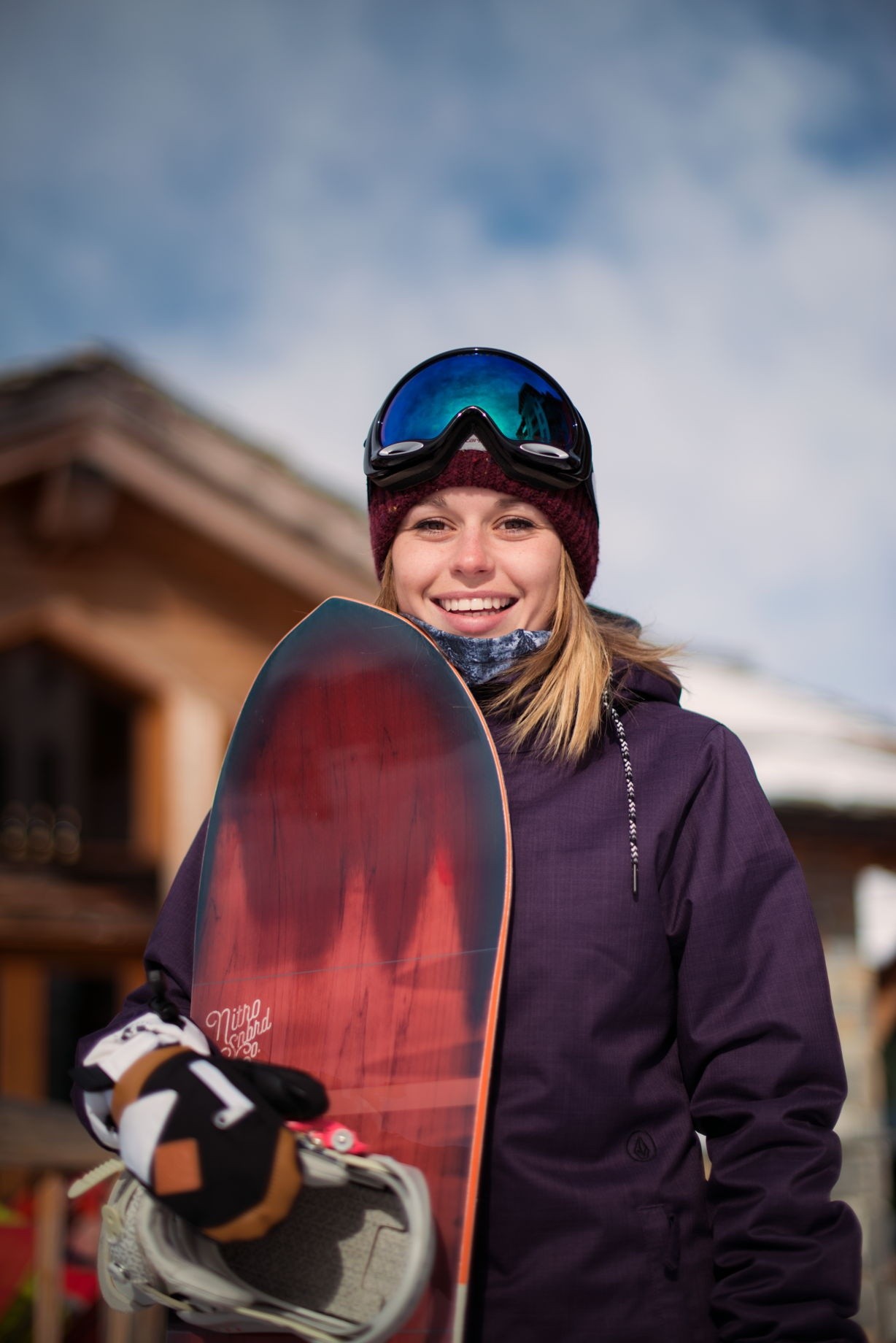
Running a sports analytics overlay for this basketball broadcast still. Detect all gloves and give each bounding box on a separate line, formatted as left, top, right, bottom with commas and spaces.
110, 1040, 330, 1245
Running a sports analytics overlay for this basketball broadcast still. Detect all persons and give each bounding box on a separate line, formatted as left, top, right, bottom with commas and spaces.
63, 347, 876, 1343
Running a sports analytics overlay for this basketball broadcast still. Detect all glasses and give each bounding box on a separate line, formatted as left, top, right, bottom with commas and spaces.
363, 345, 592, 494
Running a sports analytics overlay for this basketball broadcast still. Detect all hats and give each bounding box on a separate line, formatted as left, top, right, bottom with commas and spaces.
368, 433, 599, 601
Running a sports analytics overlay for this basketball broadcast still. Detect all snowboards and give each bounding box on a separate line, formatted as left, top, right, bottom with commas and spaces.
185, 596, 515, 1342
